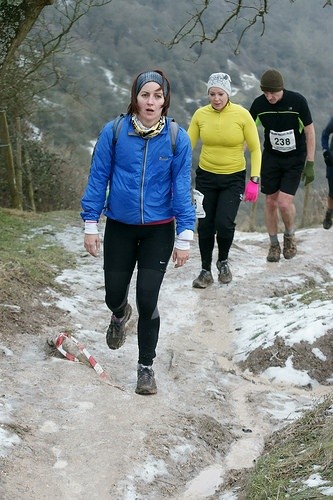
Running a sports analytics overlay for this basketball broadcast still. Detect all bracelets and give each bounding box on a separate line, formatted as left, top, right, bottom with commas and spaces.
250, 176, 260, 184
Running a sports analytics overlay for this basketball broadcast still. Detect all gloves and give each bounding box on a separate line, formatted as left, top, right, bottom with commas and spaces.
301, 161, 314, 186
323, 151, 333, 165
244, 180, 258, 203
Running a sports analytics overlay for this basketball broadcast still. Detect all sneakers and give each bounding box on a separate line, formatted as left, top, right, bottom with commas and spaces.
135, 364, 157, 394
216, 259, 232, 284
106, 304, 132, 349
267, 245, 281, 261
193, 269, 214, 288
283, 233, 297, 259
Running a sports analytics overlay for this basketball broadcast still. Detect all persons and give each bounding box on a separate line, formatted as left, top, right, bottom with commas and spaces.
184, 72, 262, 289
321, 117, 333, 229
80, 69, 196, 395
249, 69, 316, 262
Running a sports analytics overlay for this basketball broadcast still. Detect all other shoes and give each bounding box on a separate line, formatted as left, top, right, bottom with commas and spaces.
323, 211, 333, 229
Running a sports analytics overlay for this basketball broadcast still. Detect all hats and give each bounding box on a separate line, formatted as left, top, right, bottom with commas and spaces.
207, 73, 231, 99
260, 69, 283, 92
